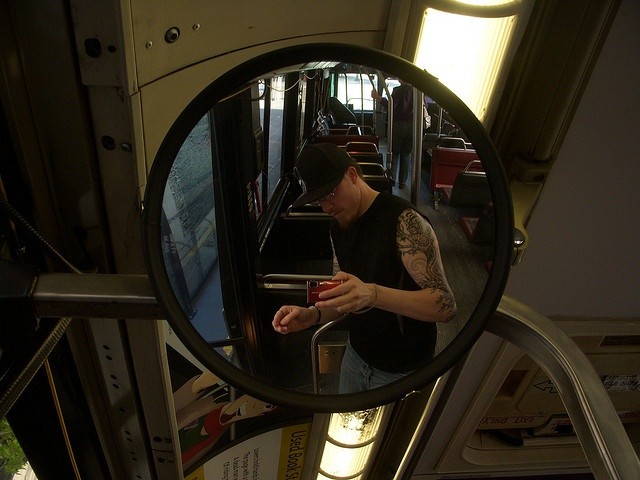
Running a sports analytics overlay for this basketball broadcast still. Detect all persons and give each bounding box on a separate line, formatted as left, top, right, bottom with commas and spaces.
368, 78, 413, 190
272, 141, 460, 395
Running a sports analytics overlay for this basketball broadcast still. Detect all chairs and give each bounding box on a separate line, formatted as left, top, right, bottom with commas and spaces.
456, 207, 492, 245
352, 174, 393, 196
345, 141, 377, 152
422, 133, 449, 150
441, 159, 491, 209
347, 150, 383, 164
343, 161, 384, 174
437, 137, 473, 149
279, 199, 334, 226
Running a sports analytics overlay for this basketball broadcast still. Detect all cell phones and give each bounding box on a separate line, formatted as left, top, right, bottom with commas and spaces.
306, 278, 354, 305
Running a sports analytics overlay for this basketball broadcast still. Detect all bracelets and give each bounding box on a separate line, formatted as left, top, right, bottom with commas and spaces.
307, 305, 322, 325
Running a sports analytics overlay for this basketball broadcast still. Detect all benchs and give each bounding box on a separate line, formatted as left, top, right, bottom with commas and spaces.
254, 263, 334, 331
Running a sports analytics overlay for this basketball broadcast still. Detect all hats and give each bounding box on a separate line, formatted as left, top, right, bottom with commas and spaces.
292, 137, 350, 210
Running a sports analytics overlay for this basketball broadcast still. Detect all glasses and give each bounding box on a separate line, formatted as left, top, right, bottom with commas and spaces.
311, 188, 336, 203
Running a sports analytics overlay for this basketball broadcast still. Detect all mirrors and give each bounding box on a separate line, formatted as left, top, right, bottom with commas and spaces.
141, 42, 514, 413
